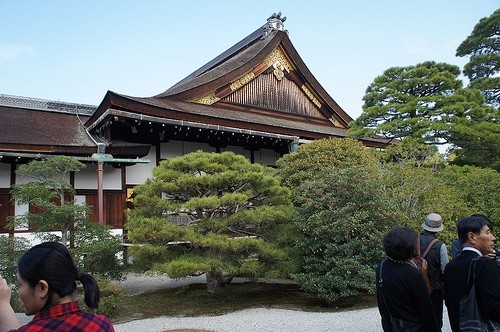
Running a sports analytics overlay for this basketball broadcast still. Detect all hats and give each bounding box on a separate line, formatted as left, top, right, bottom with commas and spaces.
421, 213, 444, 233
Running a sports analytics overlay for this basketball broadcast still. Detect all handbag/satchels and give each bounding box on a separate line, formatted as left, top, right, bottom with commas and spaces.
457, 285, 495, 332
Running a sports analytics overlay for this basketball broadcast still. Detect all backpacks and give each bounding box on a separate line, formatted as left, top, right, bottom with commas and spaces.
410, 231, 440, 294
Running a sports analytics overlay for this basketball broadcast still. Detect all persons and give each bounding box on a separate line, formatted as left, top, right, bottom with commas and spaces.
414, 213, 449, 332
375, 228, 435, 332
442, 214, 500, 332
0, 242, 115, 332
